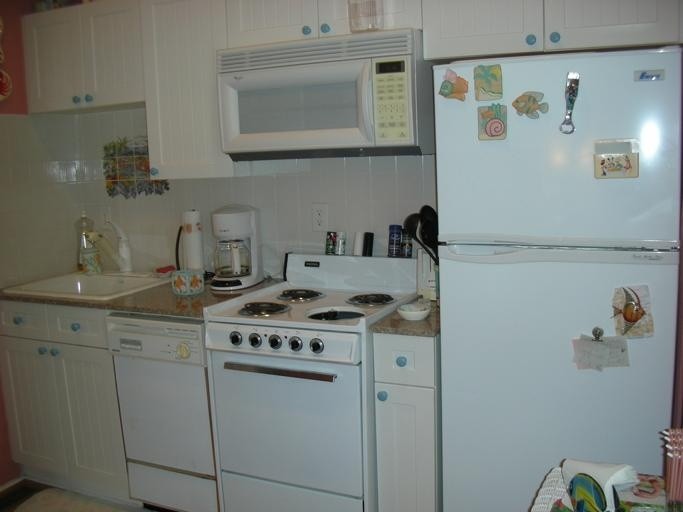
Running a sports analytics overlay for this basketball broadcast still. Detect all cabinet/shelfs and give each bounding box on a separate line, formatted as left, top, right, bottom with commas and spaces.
369, 326, 441, 512
0, 296, 142, 508
225, 0, 422, 47
421, 0, 683, 61
21, 0, 145, 115
139, 0, 309, 181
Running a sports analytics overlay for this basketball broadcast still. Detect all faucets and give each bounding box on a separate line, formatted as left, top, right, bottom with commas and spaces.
86, 220, 131, 274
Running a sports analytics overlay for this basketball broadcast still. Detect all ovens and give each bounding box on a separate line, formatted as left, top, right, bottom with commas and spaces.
206, 321, 364, 512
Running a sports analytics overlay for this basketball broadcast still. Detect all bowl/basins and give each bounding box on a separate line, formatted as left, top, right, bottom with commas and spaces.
430, 48, 681, 510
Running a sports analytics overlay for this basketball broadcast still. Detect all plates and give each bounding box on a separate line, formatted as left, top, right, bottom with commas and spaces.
396, 303, 431, 321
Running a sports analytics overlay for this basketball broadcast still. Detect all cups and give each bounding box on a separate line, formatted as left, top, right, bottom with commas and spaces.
80, 248, 102, 274
347, 0, 383, 32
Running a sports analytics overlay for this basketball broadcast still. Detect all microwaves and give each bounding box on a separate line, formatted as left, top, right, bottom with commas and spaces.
215, 31, 427, 158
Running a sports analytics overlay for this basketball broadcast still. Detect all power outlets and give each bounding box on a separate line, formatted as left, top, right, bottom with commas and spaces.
312, 204, 328, 231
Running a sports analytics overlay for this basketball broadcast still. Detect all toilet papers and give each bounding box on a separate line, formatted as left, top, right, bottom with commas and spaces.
179, 211, 203, 269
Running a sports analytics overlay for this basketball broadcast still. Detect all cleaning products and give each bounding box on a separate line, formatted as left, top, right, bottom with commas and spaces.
75, 207, 95, 270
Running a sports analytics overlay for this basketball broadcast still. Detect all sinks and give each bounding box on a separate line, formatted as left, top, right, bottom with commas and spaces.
2, 270, 173, 302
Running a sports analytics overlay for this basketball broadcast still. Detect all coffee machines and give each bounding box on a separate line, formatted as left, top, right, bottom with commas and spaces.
211, 204, 264, 289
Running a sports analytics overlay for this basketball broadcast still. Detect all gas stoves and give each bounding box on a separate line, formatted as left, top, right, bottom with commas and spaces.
205, 284, 418, 365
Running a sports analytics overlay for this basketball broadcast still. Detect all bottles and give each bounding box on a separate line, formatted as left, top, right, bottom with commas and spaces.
325, 224, 411, 258
181, 211, 204, 274
76, 210, 97, 268
